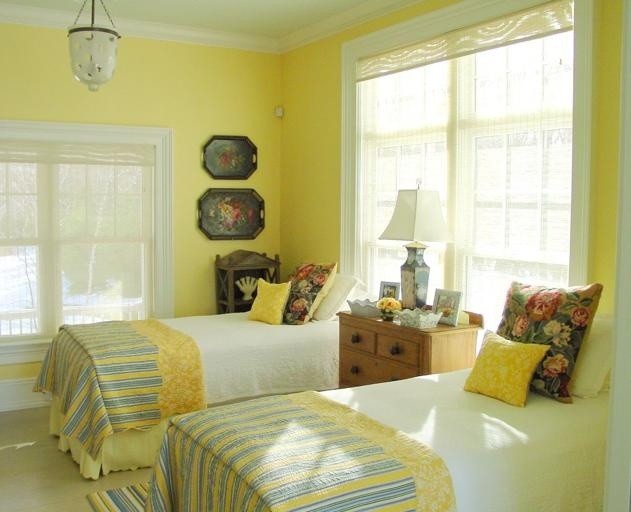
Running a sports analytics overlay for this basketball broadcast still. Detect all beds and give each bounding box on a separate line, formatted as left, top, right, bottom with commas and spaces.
145, 367, 612, 511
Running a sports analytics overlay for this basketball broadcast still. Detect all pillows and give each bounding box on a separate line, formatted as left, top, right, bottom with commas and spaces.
247, 261, 362, 324
463, 280, 617, 406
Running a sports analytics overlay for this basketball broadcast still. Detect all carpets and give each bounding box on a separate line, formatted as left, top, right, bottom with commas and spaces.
85, 481, 151, 512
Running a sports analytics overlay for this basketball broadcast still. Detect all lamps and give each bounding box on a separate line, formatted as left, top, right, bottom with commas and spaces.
68, 1, 123, 91
376, 177, 451, 309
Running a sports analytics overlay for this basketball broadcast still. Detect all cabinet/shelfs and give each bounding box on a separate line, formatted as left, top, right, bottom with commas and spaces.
214, 250, 282, 315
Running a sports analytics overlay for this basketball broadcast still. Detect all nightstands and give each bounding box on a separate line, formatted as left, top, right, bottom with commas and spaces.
335, 310, 486, 390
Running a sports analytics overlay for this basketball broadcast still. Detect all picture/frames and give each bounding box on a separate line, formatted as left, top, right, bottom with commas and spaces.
196, 136, 266, 240
377, 280, 464, 327
